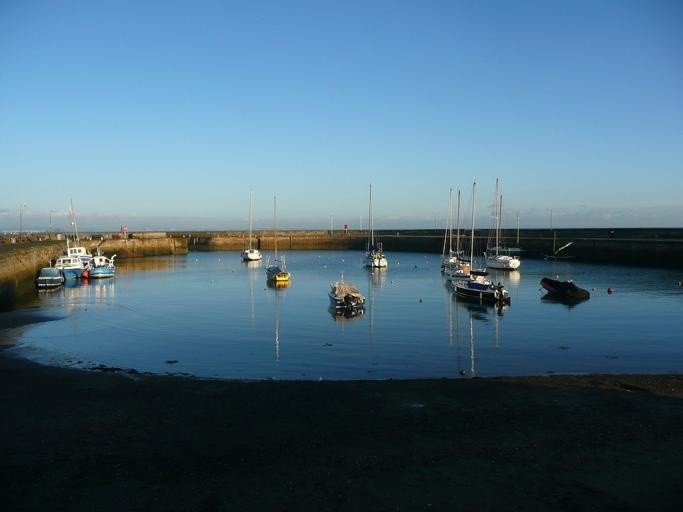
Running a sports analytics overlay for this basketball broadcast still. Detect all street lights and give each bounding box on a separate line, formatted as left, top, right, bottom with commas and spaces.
18, 202, 29, 244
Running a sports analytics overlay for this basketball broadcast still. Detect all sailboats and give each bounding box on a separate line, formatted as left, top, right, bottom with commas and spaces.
366, 266, 388, 350
362, 183, 389, 268
242, 185, 264, 261
265, 278, 291, 370
438, 175, 592, 307
444, 281, 501, 378
265, 192, 292, 281
246, 261, 261, 329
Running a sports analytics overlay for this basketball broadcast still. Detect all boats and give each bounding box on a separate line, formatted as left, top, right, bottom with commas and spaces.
327, 273, 364, 307
327, 303, 366, 331
34, 197, 118, 291
